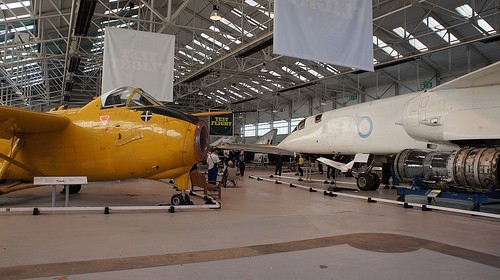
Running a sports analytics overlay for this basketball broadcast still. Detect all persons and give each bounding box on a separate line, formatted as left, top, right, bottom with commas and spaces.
206, 148, 222, 191
219, 149, 247, 189
190, 161, 203, 173
293, 153, 399, 189
275, 152, 283, 178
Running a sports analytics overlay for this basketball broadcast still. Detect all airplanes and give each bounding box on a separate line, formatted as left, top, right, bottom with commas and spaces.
209, 61, 500, 191
0, 86, 232, 205
